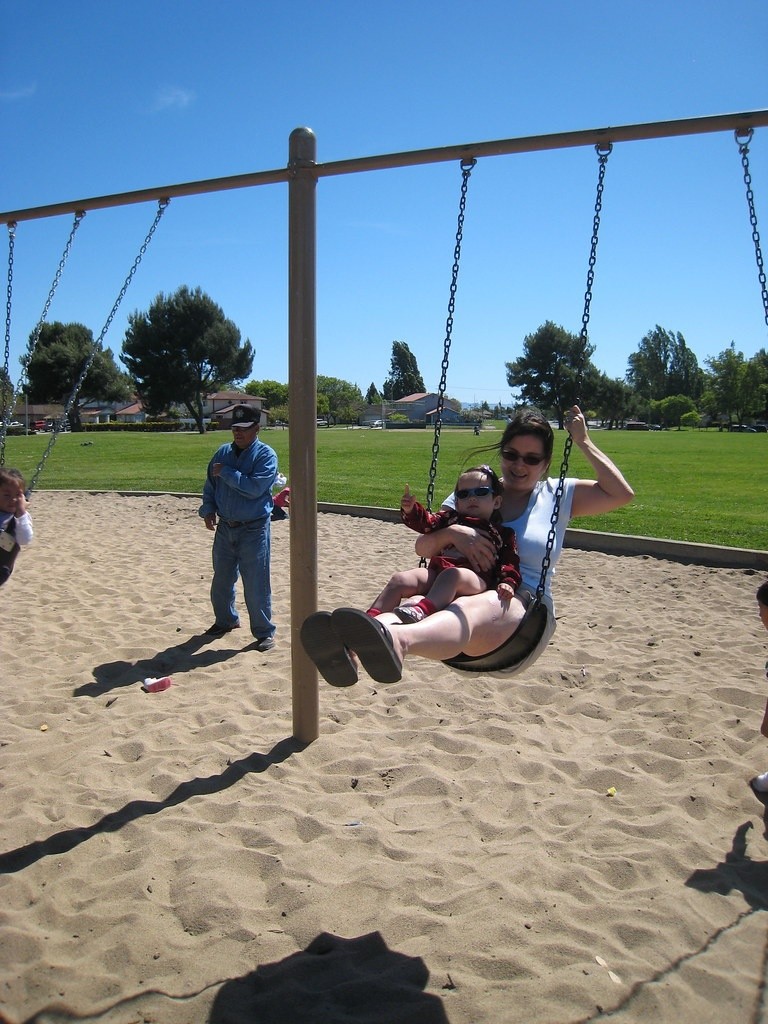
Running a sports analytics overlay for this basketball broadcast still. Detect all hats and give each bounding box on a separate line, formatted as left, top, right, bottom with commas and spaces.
232, 404, 261, 427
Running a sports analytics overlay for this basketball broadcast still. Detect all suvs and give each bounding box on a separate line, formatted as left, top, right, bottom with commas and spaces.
750, 425, 767, 432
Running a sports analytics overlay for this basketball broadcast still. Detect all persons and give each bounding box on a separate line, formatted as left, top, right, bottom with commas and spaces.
300, 405, 634, 687
198, 405, 278, 650
271, 487, 290, 521
365, 465, 522, 626
473, 427, 480, 435
507, 415, 512, 424
0, 467, 33, 586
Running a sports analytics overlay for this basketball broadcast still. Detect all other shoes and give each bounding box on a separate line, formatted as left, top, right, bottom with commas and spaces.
204, 620, 239, 638
144, 676, 171, 693
257, 636, 275, 652
752, 772, 768, 792
393, 603, 427, 624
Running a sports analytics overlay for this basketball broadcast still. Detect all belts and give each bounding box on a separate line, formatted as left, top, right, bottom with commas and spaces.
220, 516, 263, 527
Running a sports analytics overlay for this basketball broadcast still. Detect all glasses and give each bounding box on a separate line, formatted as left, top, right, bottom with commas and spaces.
502, 446, 547, 465
455, 487, 495, 500
232, 423, 256, 432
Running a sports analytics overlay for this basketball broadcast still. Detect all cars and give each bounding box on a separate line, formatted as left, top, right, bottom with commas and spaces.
370, 420, 384, 428
9, 422, 23, 427
0, 422, 8, 426
651, 425, 660, 430
732, 425, 756, 432
317, 419, 327, 426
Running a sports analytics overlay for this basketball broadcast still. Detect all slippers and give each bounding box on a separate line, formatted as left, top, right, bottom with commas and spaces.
300, 611, 359, 687
332, 607, 402, 683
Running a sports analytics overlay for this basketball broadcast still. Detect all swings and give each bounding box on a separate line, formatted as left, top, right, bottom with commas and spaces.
0, 193, 173, 573
732, 127, 768, 328
415, 139, 618, 675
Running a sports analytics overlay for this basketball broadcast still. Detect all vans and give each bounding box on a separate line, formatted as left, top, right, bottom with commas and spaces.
64, 419, 72, 430
33, 421, 47, 430
